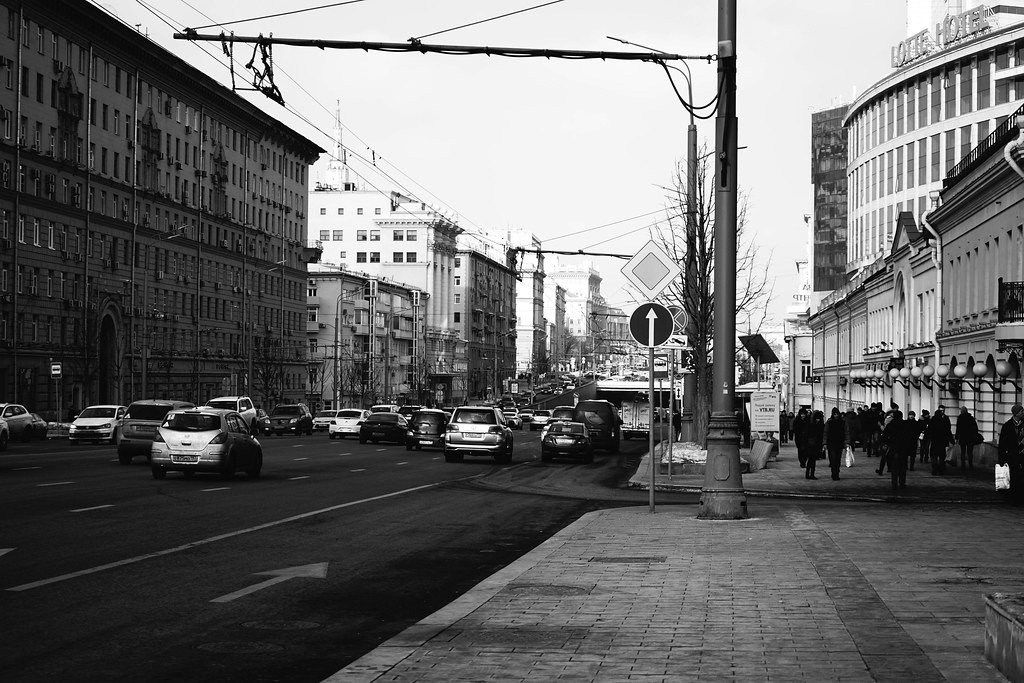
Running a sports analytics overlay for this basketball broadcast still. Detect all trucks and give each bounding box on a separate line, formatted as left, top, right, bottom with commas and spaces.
619, 397, 658, 440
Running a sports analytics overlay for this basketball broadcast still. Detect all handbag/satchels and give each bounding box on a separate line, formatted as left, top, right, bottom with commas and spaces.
994, 463, 1011, 489
943, 441, 962, 468
845, 446, 855, 467
973, 433, 984, 445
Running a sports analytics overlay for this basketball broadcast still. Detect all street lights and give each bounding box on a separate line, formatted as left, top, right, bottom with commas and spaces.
142, 224, 192, 400
332, 286, 367, 410
384, 305, 412, 405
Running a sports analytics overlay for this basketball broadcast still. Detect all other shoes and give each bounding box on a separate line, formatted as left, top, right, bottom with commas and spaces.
810, 476, 817, 480
876, 469, 882, 475
806, 472, 810, 479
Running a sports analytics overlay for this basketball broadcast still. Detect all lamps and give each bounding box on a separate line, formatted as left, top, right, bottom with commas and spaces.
806, 376, 813, 383
813, 376, 820, 383
850, 361, 1023, 393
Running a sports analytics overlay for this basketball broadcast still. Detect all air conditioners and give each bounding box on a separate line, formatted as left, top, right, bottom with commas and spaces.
1, 55, 326, 368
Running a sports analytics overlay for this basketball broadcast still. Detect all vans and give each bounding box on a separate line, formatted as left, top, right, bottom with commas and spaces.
572, 398, 624, 452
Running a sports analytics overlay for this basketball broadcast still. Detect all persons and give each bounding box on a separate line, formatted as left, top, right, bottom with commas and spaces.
998, 405, 1024, 505
879, 410, 917, 489
955, 406, 983, 470
779, 402, 954, 481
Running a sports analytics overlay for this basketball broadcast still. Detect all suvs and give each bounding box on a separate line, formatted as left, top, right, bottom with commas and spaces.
264, 402, 314, 437
204, 395, 259, 436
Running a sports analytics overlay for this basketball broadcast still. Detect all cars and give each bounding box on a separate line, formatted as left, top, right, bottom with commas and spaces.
68, 404, 130, 445
256, 409, 268, 431
440, 370, 669, 431
541, 420, 597, 464
405, 408, 449, 451
444, 405, 515, 464
0, 402, 49, 451
329, 408, 372, 439
116, 398, 198, 466
370, 404, 400, 414
395, 405, 429, 422
312, 409, 338, 432
150, 406, 263, 479
359, 412, 409, 443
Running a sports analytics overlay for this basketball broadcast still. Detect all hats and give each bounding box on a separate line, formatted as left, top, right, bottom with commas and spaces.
1011, 405, 1023, 416
959, 406, 967, 412
922, 409, 929, 415
832, 407, 839, 415
908, 411, 915, 416
